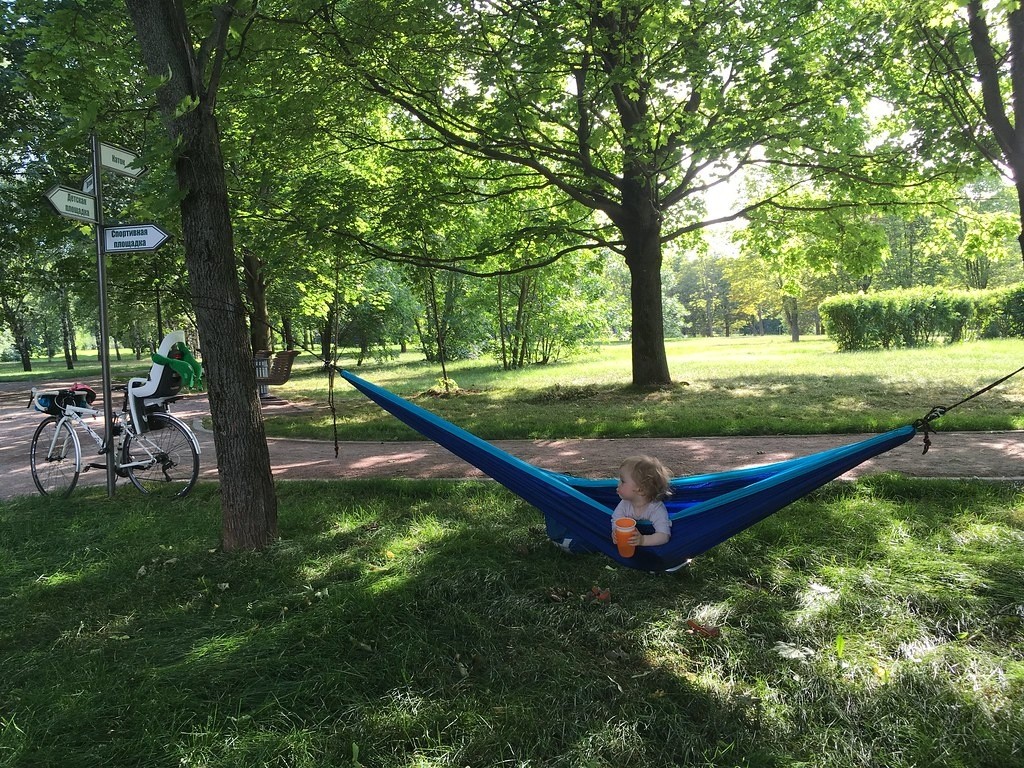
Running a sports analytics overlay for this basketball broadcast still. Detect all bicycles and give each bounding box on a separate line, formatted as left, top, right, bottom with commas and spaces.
27, 382, 201, 502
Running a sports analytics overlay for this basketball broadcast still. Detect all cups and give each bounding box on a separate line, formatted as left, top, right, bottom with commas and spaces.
615, 518, 637, 558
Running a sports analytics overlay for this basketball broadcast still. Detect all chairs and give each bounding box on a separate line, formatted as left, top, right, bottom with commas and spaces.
127, 330, 187, 435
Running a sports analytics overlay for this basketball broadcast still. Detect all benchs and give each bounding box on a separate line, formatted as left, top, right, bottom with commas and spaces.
252, 350, 302, 399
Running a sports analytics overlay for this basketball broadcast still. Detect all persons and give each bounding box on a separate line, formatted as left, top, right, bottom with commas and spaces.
610, 453, 674, 547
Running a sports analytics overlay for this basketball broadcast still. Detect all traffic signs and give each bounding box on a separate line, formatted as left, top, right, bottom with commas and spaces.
42, 134, 173, 255
